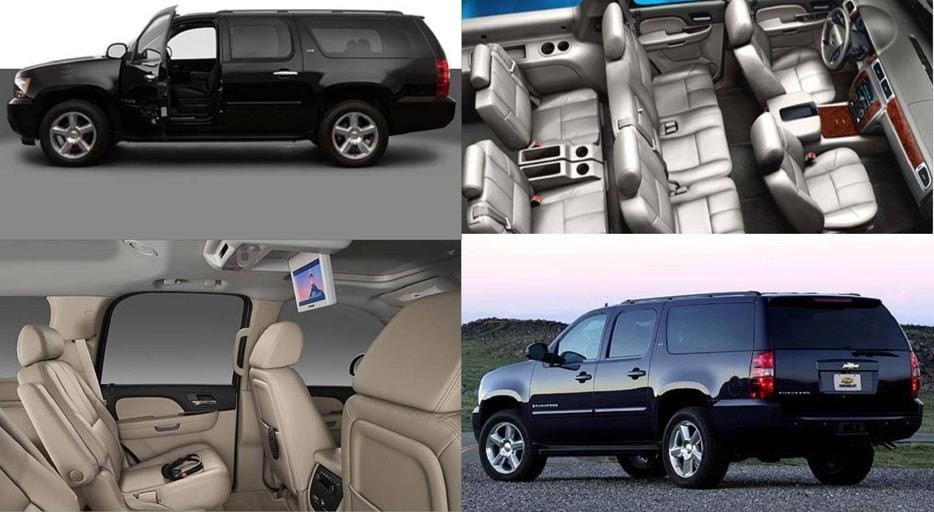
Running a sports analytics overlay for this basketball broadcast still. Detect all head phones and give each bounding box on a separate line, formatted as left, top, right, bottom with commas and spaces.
160, 453, 203, 480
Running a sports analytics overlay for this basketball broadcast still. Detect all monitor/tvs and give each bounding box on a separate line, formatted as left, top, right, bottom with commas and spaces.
288, 251, 337, 314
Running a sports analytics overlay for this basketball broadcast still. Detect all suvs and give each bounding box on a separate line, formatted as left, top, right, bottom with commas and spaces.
473, 286, 925, 491
7, 3, 458, 168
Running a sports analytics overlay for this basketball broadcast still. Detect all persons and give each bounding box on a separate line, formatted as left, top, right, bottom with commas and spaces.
307, 273, 324, 299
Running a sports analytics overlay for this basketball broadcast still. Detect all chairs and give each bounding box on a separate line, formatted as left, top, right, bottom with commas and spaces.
176, 58, 219, 110
460, 0, 878, 234
0, 290, 461, 512
